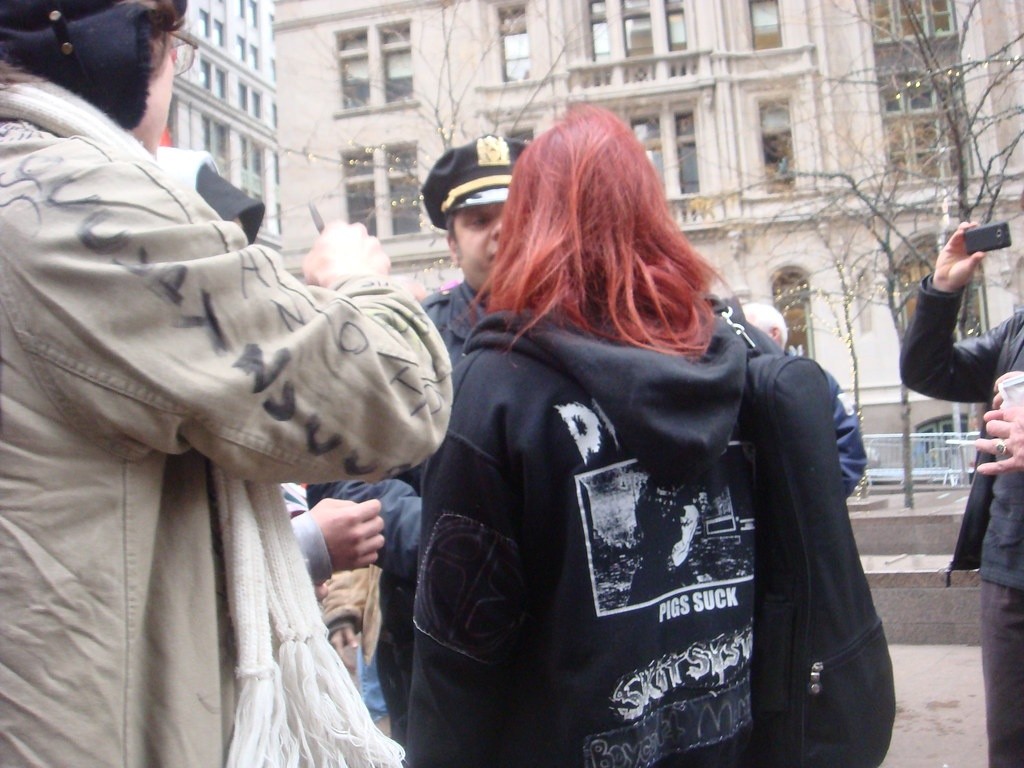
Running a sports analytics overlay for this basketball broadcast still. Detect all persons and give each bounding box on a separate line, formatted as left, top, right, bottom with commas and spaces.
976, 371, 1024, 475
744, 301, 869, 499
0, 0, 779, 768
900, 222, 1024, 768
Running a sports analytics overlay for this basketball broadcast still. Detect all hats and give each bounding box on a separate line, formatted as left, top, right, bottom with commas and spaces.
0, 0, 189, 129
195, 165, 266, 245
422, 136, 526, 229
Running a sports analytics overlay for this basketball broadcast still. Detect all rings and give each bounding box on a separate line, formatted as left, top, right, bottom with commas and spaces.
995, 439, 1008, 456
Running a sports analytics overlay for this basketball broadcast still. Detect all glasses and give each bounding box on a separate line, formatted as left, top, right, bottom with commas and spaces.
149, 20, 198, 75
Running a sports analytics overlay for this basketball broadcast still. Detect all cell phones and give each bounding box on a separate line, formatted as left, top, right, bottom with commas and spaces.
965, 221, 1011, 256
998, 374, 1024, 405
